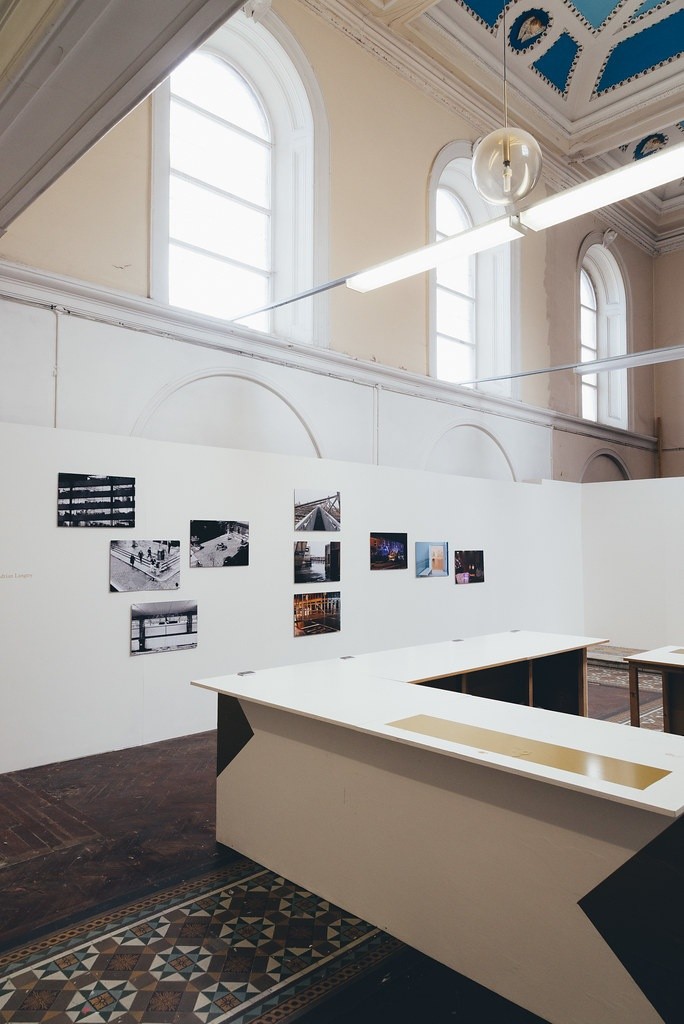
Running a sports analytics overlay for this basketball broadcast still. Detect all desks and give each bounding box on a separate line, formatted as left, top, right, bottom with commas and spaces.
192, 626, 682, 1022
623, 641, 683, 737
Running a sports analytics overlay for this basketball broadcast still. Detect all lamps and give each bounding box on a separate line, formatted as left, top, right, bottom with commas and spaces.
470, 3, 544, 207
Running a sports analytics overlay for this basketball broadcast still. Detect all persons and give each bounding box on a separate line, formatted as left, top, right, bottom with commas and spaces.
216, 525, 245, 550
130, 547, 161, 577
